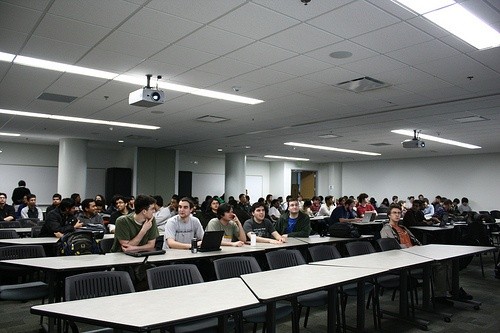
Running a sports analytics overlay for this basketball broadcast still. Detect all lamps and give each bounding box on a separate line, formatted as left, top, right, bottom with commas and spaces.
390, 0, 500, 52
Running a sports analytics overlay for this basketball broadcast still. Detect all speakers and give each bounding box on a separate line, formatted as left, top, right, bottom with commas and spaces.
179, 170, 192, 198
105, 167, 131, 204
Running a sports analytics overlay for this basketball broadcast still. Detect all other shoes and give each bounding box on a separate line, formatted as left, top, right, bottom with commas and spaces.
434, 296, 454, 306
457, 290, 473, 300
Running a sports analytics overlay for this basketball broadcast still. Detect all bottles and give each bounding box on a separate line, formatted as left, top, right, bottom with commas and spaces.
251, 232, 257, 246
191, 238, 198, 253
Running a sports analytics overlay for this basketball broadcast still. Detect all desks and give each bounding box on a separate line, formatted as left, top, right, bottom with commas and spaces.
409, 226, 454, 245
433, 219, 500, 225
0, 219, 500, 333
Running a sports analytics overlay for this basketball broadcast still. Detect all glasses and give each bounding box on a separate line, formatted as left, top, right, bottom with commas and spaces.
392, 211, 402, 215
289, 203, 299, 207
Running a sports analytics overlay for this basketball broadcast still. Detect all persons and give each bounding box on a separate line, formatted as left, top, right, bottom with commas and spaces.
257, 195, 292, 225
71, 192, 136, 234
12, 180, 30, 212
47, 193, 62, 216
328, 199, 360, 225
16, 194, 43, 221
299, 193, 399, 219
380, 204, 473, 309
401, 195, 471, 226
40, 198, 84, 237
198, 194, 252, 229
275, 198, 311, 238
150, 194, 180, 225
243, 202, 286, 245
205, 203, 246, 246
109, 194, 159, 283
0, 193, 16, 221
163, 198, 205, 265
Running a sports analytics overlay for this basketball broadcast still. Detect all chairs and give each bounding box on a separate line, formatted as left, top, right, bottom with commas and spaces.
145, 264, 218, 333
345, 241, 401, 328
213, 256, 295, 333
65, 271, 135, 333
308, 245, 375, 330
265, 249, 328, 329
0, 244, 48, 325
0, 230, 17, 239
0, 221, 21, 228
376, 237, 422, 318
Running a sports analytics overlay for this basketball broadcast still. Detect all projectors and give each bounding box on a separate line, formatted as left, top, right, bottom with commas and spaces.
402, 140, 426, 148
128, 89, 166, 107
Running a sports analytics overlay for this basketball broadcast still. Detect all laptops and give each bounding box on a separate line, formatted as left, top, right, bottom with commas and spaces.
433, 214, 448, 227
126, 248, 165, 257
355, 211, 373, 222
190, 230, 225, 251
